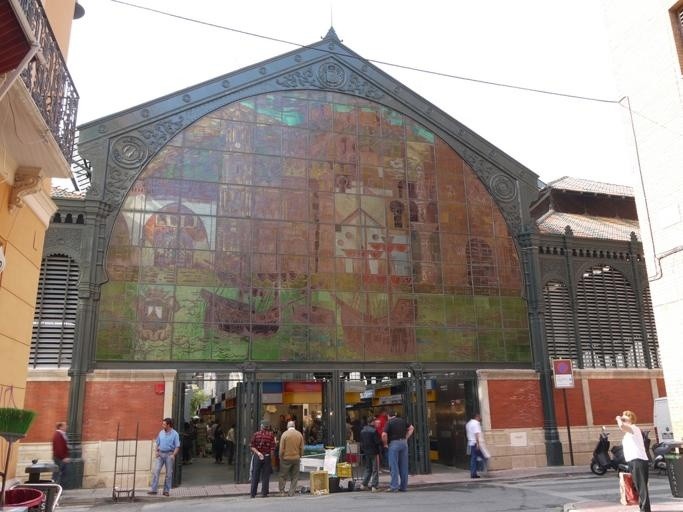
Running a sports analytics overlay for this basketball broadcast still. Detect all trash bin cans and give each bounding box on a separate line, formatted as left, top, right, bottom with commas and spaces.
3, 460, 63, 512
664, 453, 682, 498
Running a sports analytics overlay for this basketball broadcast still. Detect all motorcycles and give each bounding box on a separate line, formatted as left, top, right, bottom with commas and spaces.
588, 427, 631, 476
640, 427, 674, 475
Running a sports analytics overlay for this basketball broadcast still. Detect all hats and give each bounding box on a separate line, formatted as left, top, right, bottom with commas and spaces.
261, 419, 270, 426
366, 416, 375, 423
287, 420, 295, 426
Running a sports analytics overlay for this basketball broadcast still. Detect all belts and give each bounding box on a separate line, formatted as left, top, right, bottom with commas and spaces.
265, 453, 270, 456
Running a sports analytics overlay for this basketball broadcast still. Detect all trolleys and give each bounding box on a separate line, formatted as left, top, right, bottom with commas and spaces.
110, 419, 147, 510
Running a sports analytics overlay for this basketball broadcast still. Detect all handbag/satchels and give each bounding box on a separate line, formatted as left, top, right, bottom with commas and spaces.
223, 447, 231, 457
618, 472, 639, 506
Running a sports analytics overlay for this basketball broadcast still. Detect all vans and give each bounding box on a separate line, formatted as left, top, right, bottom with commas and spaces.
649, 395, 682, 453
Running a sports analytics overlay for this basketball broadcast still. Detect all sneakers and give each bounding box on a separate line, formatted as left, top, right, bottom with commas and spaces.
250, 493, 267, 498
162, 491, 168, 496
146, 491, 157, 494
359, 483, 408, 492
214, 461, 234, 465
470, 475, 480, 478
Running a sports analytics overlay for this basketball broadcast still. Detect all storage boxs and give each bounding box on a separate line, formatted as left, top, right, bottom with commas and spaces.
336, 462, 353, 477
329, 477, 355, 493
309, 470, 329, 496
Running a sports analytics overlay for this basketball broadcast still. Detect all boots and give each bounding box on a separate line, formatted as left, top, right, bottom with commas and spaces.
274, 489, 294, 496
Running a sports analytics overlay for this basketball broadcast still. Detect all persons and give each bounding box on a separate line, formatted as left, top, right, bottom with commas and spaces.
146, 417, 180, 496
180, 407, 415, 499
464, 410, 494, 478
616, 411, 651, 512
52, 422, 71, 490
